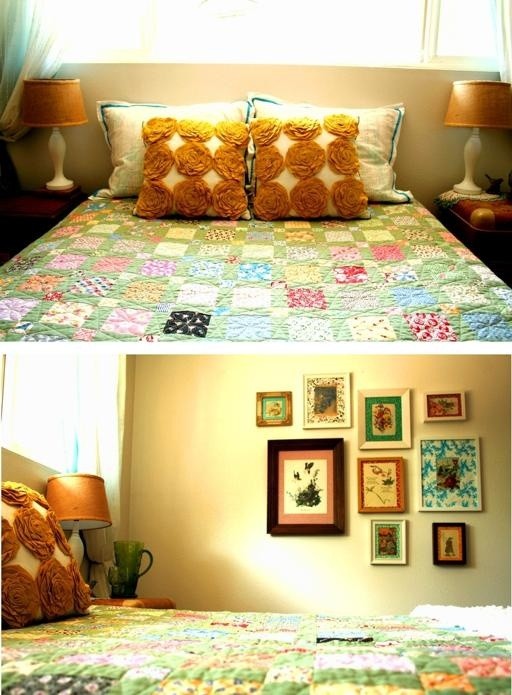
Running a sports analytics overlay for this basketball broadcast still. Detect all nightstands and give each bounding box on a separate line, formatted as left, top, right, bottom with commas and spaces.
0, 193, 90, 267
91, 598, 176, 610
446, 198, 512, 289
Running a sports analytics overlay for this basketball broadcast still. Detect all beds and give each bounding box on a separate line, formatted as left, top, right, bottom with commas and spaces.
1, 447, 511, 695
0, 63, 512, 342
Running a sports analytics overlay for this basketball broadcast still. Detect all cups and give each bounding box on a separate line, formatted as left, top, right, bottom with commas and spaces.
108, 538, 155, 599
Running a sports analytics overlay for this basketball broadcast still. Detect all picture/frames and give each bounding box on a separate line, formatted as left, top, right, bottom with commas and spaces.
357, 388, 412, 449
432, 522, 467, 565
370, 519, 407, 565
416, 435, 483, 513
357, 457, 406, 513
267, 438, 345, 534
256, 391, 293, 426
424, 392, 466, 421
303, 372, 351, 428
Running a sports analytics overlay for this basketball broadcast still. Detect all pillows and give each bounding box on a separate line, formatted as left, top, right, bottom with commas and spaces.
248, 91, 413, 204
87, 101, 253, 201
1, 481, 91, 628
132, 115, 251, 221
248, 114, 372, 221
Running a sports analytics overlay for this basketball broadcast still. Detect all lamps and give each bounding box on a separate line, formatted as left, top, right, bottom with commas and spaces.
45, 474, 112, 570
16, 77, 89, 200
443, 80, 512, 196
